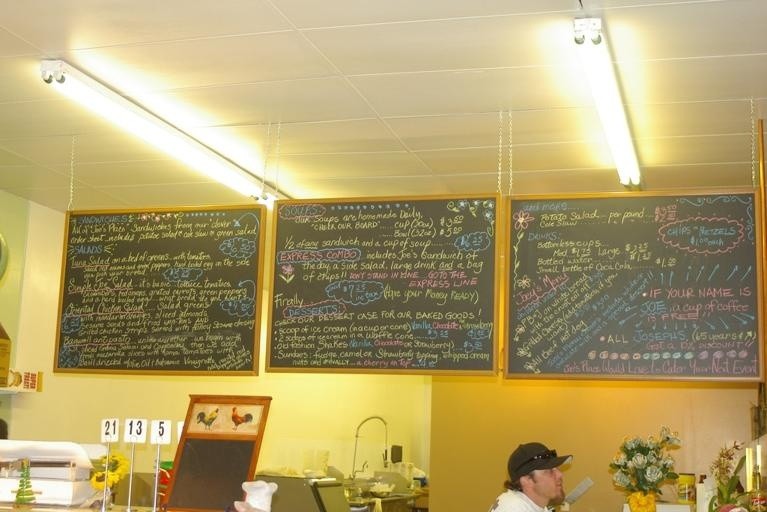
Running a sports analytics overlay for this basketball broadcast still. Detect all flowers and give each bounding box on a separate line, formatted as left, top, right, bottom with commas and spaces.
708, 439, 757, 512
606, 424, 683, 498
88, 452, 130, 491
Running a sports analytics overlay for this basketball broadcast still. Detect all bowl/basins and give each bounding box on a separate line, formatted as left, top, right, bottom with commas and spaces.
370, 491, 390, 498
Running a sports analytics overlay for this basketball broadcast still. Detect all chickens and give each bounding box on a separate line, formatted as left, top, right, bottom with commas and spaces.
197, 408, 219, 431
232, 407, 253, 431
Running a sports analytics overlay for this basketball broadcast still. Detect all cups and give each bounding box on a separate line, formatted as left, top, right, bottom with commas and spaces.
412, 480, 421, 490
303, 449, 330, 478
744, 446, 767, 512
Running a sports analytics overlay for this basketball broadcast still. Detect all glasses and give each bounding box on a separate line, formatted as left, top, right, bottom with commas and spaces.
514, 449, 557, 469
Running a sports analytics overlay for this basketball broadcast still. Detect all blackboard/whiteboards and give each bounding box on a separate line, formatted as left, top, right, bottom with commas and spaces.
54, 203, 266, 378
261, 193, 504, 379
162, 434, 263, 512
505, 189, 761, 378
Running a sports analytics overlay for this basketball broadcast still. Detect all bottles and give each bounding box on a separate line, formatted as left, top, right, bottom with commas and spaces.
13, 457, 36, 510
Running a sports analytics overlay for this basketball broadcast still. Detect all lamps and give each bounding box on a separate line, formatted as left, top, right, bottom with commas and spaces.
38, 58, 290, 211
573, 16, 646, 190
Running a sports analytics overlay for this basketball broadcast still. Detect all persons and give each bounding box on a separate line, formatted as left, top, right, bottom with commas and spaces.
482, 442, 571, 512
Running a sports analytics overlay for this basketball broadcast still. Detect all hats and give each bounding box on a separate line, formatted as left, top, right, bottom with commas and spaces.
506, 440, 574, 483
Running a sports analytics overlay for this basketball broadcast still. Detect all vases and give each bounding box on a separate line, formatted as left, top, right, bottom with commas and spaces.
626, 490, 656, 512
